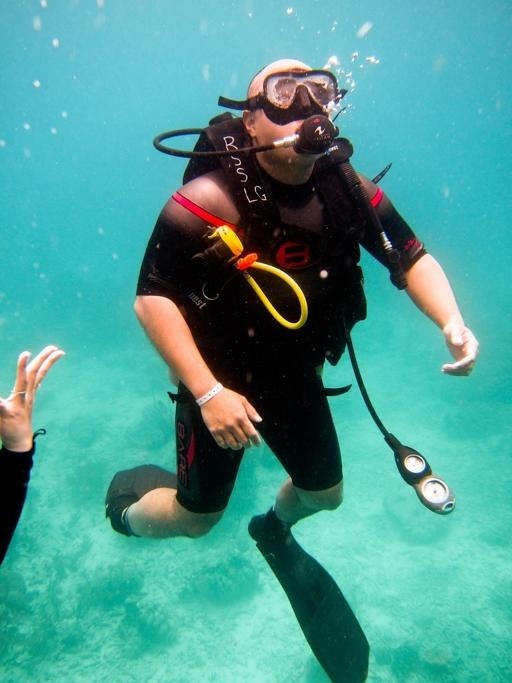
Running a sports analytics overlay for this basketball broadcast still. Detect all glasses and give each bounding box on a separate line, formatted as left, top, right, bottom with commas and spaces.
260, 70, 338, 112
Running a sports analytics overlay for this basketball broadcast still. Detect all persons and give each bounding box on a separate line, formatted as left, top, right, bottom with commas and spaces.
0, 339, 66, 584
100, 50, 482, 683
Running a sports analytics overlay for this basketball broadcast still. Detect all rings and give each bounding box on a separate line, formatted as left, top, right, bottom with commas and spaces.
12, 388, 28, 396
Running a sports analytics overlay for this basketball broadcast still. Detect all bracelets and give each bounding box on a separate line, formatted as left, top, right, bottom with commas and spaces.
191, 380, 224, 409
